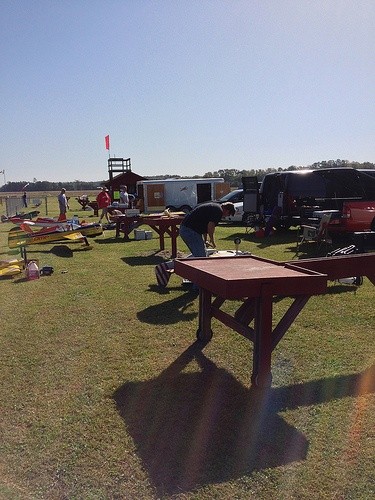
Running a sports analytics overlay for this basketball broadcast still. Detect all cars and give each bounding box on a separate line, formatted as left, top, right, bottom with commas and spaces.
213, 188, 243, 227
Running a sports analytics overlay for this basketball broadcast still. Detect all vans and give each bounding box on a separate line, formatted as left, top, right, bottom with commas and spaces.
242, 166, 375, 236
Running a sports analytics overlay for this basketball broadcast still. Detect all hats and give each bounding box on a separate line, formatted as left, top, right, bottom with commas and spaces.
101, 187, 109, 191
222, 202, 235, 214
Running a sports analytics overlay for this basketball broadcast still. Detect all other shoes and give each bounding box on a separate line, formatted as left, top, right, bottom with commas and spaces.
108, 221, 111, 224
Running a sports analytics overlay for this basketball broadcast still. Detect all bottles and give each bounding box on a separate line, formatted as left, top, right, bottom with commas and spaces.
73, 214, 79, 230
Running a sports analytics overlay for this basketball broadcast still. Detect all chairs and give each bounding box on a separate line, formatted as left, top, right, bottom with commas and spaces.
296, 213, 335, 255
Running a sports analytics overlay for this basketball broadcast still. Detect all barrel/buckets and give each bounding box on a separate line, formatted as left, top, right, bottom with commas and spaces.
26, 261, 39, 281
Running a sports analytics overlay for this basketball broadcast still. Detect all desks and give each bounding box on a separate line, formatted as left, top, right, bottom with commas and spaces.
88, 201, 186, 257
